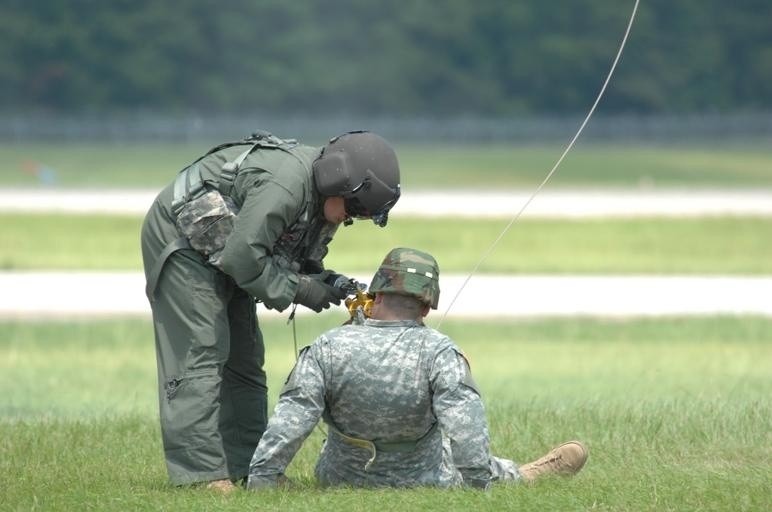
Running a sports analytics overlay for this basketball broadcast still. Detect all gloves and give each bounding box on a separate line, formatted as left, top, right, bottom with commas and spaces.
294, 270, 345, 311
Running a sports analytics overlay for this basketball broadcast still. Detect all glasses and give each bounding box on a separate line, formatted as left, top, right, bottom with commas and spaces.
343, 195, 365, 217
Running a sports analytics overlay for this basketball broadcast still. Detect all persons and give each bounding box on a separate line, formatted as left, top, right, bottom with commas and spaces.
138, 130, 404, 492
240, 245, 590, 491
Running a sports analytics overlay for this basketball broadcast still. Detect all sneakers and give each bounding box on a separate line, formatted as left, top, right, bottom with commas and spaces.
197, 478, 237, 495
520, 441, 587, 488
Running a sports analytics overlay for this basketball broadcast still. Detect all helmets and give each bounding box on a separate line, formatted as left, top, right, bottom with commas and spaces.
314, 130, 400, 214
370, 247, 441, 309
